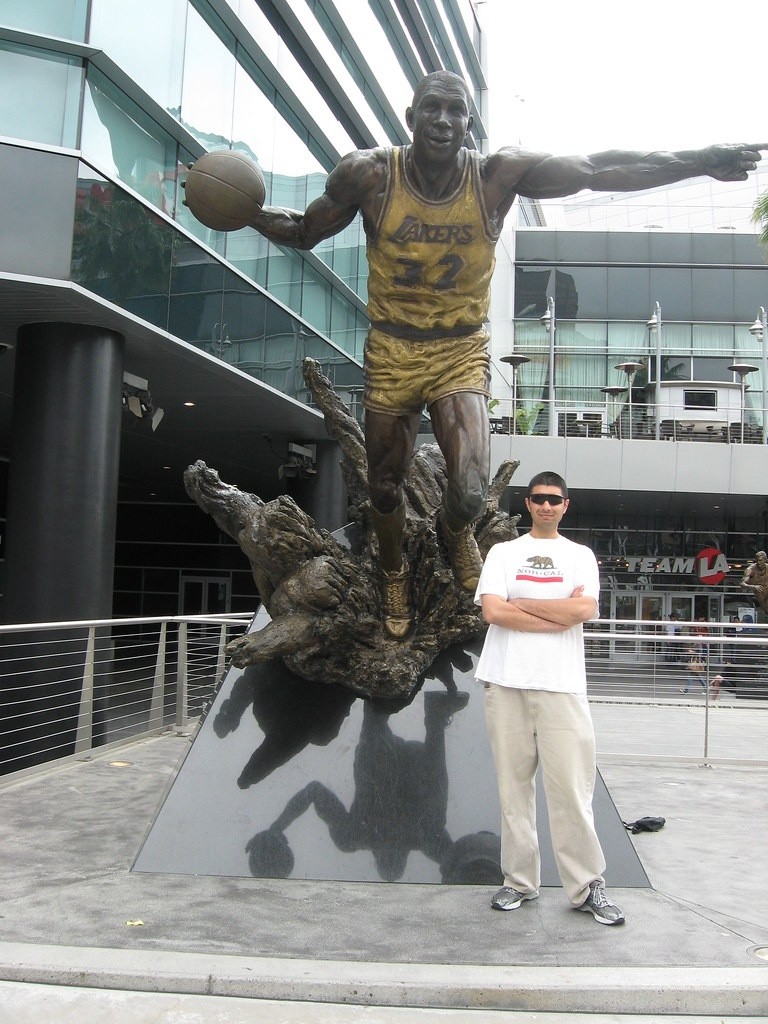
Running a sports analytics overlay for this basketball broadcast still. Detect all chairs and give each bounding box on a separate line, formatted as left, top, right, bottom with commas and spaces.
661, 419, 680, 441
683, 423, 695, 441
706, 425, 722, 442
730, 422, 749, 443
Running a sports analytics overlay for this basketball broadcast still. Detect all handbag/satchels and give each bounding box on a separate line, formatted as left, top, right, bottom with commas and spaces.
622, 816, 665, 834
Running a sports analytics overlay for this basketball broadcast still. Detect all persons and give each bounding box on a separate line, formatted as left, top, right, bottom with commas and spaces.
665, 613, 756, 700
180, 71, 768, 641
646, 610, 662, 654
467, 471, 622, 926
741, 551, 768, 614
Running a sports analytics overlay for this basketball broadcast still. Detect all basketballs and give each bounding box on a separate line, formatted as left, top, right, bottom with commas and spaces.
185, 149, 265, 234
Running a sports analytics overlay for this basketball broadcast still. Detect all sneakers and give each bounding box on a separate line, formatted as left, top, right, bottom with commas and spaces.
578, 881, 625, 926
490, 886, 539, 910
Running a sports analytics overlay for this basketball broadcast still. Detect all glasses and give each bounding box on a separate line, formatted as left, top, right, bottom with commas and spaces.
528, 493, 567, 505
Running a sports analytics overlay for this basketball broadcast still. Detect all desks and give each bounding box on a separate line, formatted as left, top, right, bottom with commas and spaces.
574, 419, 602, 437
